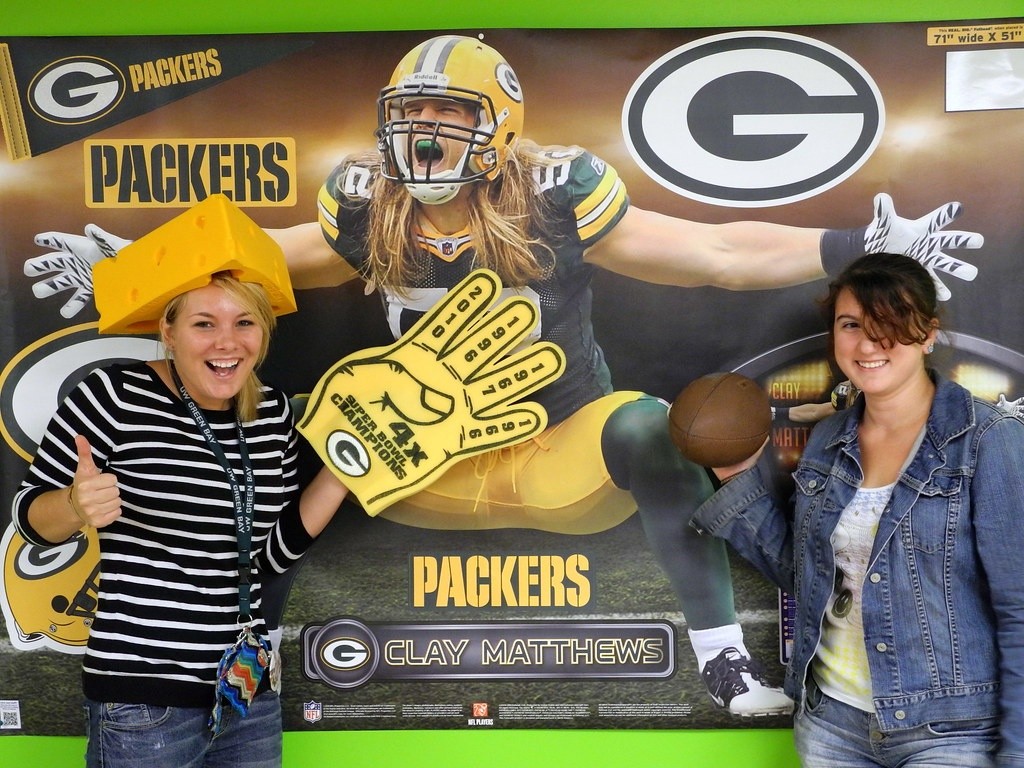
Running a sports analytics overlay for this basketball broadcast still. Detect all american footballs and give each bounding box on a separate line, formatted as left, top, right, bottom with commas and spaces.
668, 371, 772, 468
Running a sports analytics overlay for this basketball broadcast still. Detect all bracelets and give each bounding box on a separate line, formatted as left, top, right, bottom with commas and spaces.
68, 484, 85, 522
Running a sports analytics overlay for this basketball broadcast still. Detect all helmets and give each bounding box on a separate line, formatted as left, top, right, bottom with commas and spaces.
385, 34, 524, 181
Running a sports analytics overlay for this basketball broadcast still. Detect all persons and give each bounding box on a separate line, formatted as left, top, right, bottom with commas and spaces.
24, 34, 985, 722
11, 260, 350, 768
688, 252, 1024, 768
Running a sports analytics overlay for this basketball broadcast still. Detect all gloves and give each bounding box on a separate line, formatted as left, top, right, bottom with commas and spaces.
820, 191, 984, 302
24, 223, 133, 319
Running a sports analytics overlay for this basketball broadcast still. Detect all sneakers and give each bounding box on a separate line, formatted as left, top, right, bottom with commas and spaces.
701, 647, 794, 716
